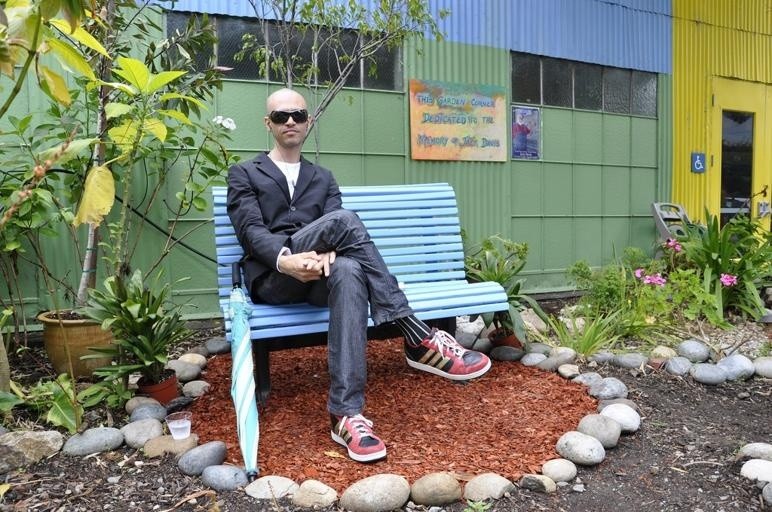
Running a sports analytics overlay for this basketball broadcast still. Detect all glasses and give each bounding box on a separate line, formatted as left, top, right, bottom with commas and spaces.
271, 109, 308, 123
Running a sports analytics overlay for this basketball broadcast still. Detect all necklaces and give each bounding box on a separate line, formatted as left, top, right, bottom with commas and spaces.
275, 142, 300, 188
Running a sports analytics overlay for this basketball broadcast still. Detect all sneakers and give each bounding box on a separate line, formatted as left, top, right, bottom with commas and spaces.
330, 413, 387, 462
404, 327, 492, 381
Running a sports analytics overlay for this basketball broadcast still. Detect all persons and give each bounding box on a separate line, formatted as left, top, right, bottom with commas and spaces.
226, 88, 492, 463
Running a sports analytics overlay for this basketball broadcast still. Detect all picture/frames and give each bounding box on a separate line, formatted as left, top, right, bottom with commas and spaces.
512, 106, 541, 159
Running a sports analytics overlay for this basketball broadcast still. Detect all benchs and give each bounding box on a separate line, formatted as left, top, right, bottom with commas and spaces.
212, 182, 510, 408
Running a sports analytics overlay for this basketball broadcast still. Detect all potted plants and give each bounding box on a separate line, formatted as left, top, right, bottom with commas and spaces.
464, 234, 527, 350
78, 262, 199, 410
36, 0, 235, 378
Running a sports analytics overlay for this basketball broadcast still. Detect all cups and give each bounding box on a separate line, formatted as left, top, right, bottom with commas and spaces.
164, 410, 195, 441
